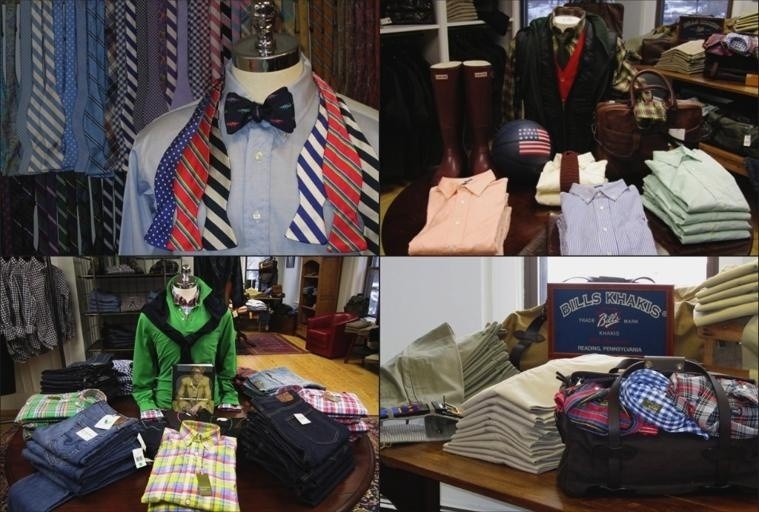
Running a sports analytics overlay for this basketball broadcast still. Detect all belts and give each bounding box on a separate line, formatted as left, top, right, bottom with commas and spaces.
380, 395, 463, 419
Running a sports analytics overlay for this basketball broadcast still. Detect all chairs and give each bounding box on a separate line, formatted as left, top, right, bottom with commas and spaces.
305, 311, 359, 360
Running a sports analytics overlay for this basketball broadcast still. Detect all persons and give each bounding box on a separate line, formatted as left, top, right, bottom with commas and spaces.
504, 6, 651, 158
177, 366, 215, 420
132, 264, 240, 420
117, 0, 380, 255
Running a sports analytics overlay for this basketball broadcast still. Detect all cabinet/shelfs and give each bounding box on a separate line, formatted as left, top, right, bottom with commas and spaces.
379, 0, 522, 81
630, 64, 759, 178
50, 256, 101, 368
77, 256, 182, 352
295, 256, 342, 338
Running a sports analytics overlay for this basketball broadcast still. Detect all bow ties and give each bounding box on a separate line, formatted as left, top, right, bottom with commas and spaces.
224, 85, 298, 133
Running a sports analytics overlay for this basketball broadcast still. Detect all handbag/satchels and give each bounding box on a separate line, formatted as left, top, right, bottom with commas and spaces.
553, 348, 758, 496
593, 70, 704, 193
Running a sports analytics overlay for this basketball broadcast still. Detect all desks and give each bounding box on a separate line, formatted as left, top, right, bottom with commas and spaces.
381, 144, 754, 256
379, 350, 759, 512
244, 295, 281, 332
5, 392, 376, 512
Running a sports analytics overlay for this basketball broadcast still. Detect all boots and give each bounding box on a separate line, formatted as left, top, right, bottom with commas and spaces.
462, 60, 497, 178
431, 60, 461, 185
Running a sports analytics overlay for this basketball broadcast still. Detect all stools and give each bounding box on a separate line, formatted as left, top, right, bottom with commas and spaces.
344, 335, 369, 366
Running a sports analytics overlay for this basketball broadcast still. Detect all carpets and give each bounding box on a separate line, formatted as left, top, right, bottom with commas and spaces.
237, 332, 307, 355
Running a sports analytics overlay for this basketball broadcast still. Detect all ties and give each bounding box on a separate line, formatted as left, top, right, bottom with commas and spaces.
3, 2, 224, 254
554, 28, 572, 70
560, 151, 580, 193
143, 69, 379, 254
